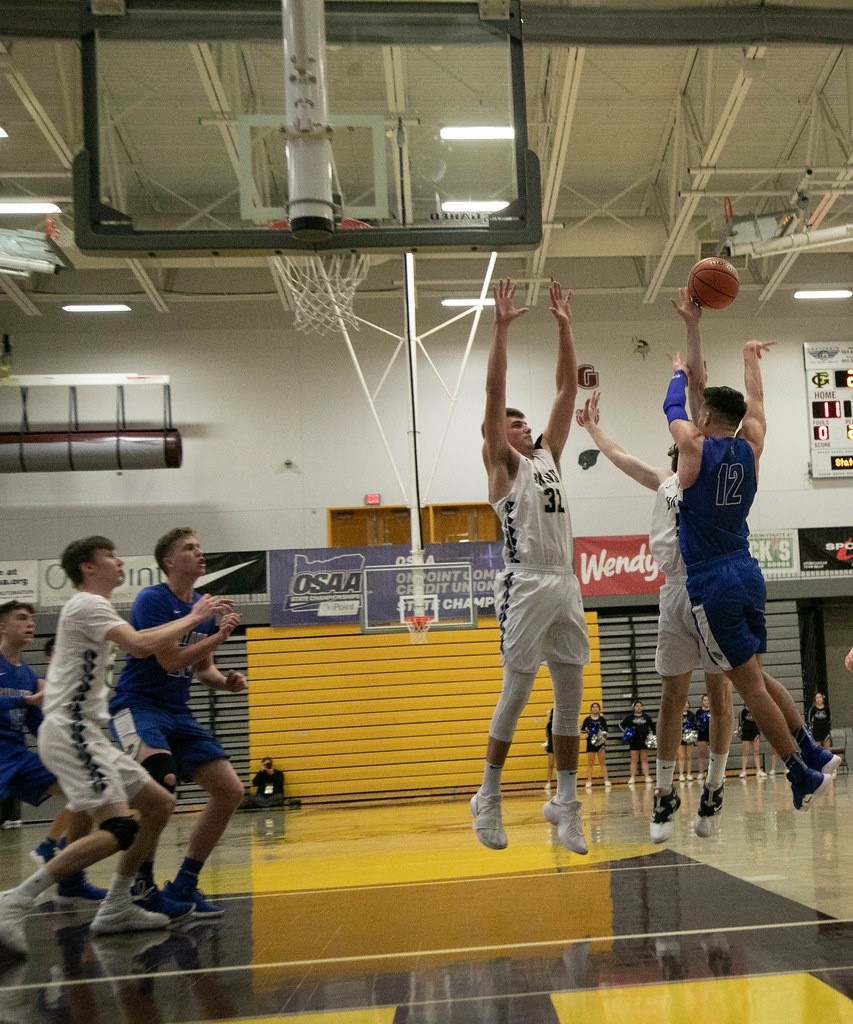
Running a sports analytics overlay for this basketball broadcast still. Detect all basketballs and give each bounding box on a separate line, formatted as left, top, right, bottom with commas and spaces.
687, 256, 740, 310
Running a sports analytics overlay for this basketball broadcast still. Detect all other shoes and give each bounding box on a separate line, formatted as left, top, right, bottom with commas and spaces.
740, 771, 746, 778
545, 776, 653, 790
697, 774, 703, 779
757, 770, 767, 776
686, 774, 693, 780
831, 771, 837, 780
785, 768, 790, 774
679, 775, 685, 781
770, 769, 775, 776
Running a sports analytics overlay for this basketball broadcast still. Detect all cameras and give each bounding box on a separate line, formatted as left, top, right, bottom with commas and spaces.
264, 762, 273, 769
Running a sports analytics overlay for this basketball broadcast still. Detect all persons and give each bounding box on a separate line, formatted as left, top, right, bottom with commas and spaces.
662, 340, 843, 811
695, 694, 726, 780
0, 525, 249, 961
544, 708, 555, 790
770, 745, 790, 775
678, 699, 695, 782
470, 279, 590, 855
618, 701, 656, 783
583, 284, 733, 844
251, 757, 284, 807
580, 703, 612, 787
807, 692, 836, 774
738, 701, 767, 777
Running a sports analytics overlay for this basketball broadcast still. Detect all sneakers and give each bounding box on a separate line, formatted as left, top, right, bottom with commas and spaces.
89, 904, 170, 933
131, 883, 196, 922
786, 768, 833, 812
29, 837, 67, 868
89, 934, 199, 978
543, 794, 589, 855
56, 879, 110, 900
693, 777, 726, 837
471, 786, 508, 850
162, 882, 225, 917
801, 744, 842, 781
0, 890, 37, 954
650, 785, 682, 843
